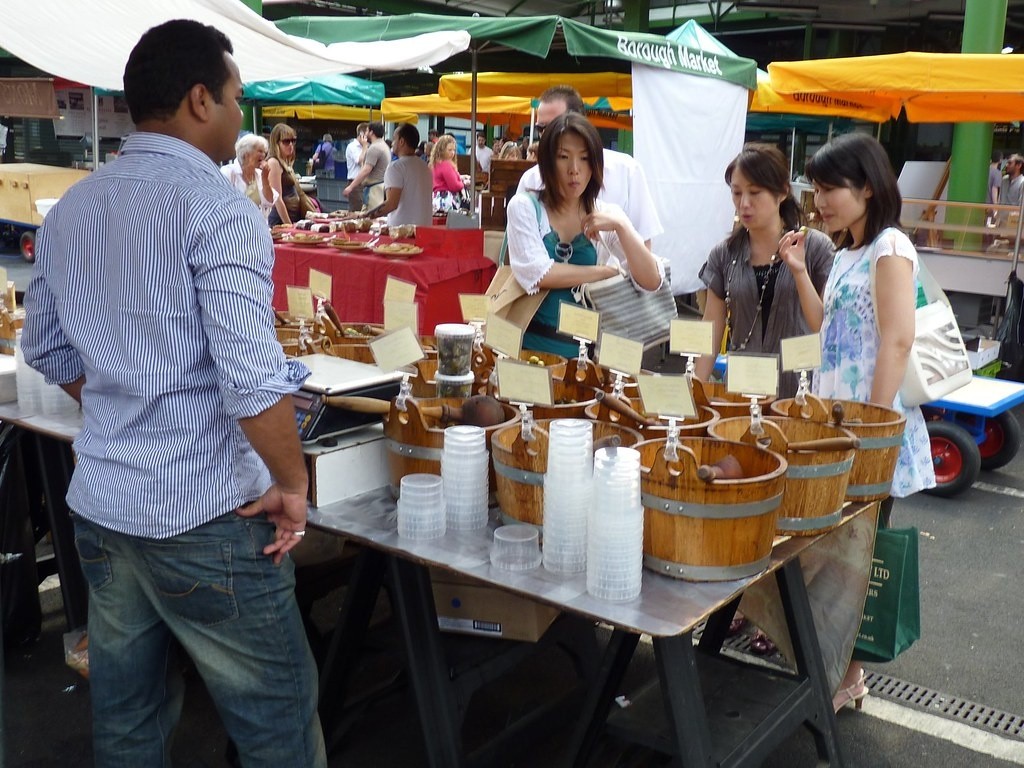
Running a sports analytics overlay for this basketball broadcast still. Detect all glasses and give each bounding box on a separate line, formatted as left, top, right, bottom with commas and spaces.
535, 125, 547, 131
278, 139, 296, 145
555, 241, 573, 264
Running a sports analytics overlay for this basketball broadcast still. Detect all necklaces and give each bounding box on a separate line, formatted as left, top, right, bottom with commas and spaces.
725, 248, 776, 351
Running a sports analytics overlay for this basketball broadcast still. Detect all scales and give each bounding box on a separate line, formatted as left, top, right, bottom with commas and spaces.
286, 354, 403, 445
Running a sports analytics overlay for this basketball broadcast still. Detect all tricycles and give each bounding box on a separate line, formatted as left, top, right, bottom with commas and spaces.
920, 376, 1023, 497
1, 163, 94, 262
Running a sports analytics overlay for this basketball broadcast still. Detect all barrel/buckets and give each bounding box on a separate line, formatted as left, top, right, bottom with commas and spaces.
629, 437, 788, 581
585, 397, 720, 440
707, 416, 857, 536
274, 311, 778, 419
383, 396, 521, 499
770, 398, 907, 503
491, 419, 644, 542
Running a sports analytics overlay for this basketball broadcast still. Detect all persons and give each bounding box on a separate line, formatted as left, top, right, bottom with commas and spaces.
507, 112, 665, 358
983, 148, 1024, 246
778, 127, 937, 714
19, 17, 331, 768
516, 83, 665, 251
519, 127, 539, 161
428, 134, 470, 215
312, 134, 336, 179
342, 120, 391, 211
693, 142, 838, 656
468, 132, 493, 172
346, 122, 370, 212
219, 132, 279, 224
417, 141, 434, 165
492, 136, 523, 160
446, 132, 466, 155
341, 122, 433, 227
264, 123, 301, 227
429, 128, 438, 144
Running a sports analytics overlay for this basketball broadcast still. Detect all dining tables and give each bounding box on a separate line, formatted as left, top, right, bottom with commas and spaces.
268, 225, 498, 337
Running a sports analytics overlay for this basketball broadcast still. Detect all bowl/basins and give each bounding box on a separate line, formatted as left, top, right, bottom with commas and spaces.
16, 328, 80, 416
397, 419, 644, 600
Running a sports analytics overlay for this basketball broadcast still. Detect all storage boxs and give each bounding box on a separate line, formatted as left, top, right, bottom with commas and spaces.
428, 583, 562, 644
428, 563, 493, 588
315, 177, 349, 212
973, 358, 1002, 376
964, 339, 1001, 371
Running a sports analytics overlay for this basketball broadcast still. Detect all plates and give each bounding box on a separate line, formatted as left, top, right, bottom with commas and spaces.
372, 248, 423, 259
327, 243, 376, 249
287, 238, 330, 247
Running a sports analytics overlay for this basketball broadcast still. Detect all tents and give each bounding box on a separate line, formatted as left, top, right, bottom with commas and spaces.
270, 14, 891, 140
769, 51, 1024, 123
263, 104, 418, 140
744, 111, 854, 182
238, 73, 385, 124
1, 1, 471, 93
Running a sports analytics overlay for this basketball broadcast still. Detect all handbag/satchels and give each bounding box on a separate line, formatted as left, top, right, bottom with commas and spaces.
313, 144, 326, 169
453, 187, 471, 210
481, 191, 552, 336
870, 227, 972, 408
300, 192, 316, 218
571, 202, 678, 358
851, 509, 920, 663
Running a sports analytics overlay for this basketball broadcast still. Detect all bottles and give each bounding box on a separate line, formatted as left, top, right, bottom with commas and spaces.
434, 323, 475, 375
434, 369, 475, 398
306, 161, 312, 175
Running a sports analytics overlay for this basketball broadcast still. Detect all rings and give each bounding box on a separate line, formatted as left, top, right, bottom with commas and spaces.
294, 531, 306, 536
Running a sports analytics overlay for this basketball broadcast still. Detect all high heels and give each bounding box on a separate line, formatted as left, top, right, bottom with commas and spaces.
833, 668, 869, 715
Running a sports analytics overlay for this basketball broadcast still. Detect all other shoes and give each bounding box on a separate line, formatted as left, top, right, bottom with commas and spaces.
725, 615, 746, 638
750, 629, 777, 655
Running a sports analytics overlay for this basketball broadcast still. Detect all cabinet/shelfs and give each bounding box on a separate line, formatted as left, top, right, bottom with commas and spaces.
901, 197, 1024, 263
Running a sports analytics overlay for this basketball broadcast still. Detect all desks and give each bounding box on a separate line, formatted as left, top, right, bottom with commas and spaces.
0, 352, 79, 642
20, 395, 116, 633
286, 485, 880, 768
918, 253, 1024, 340
